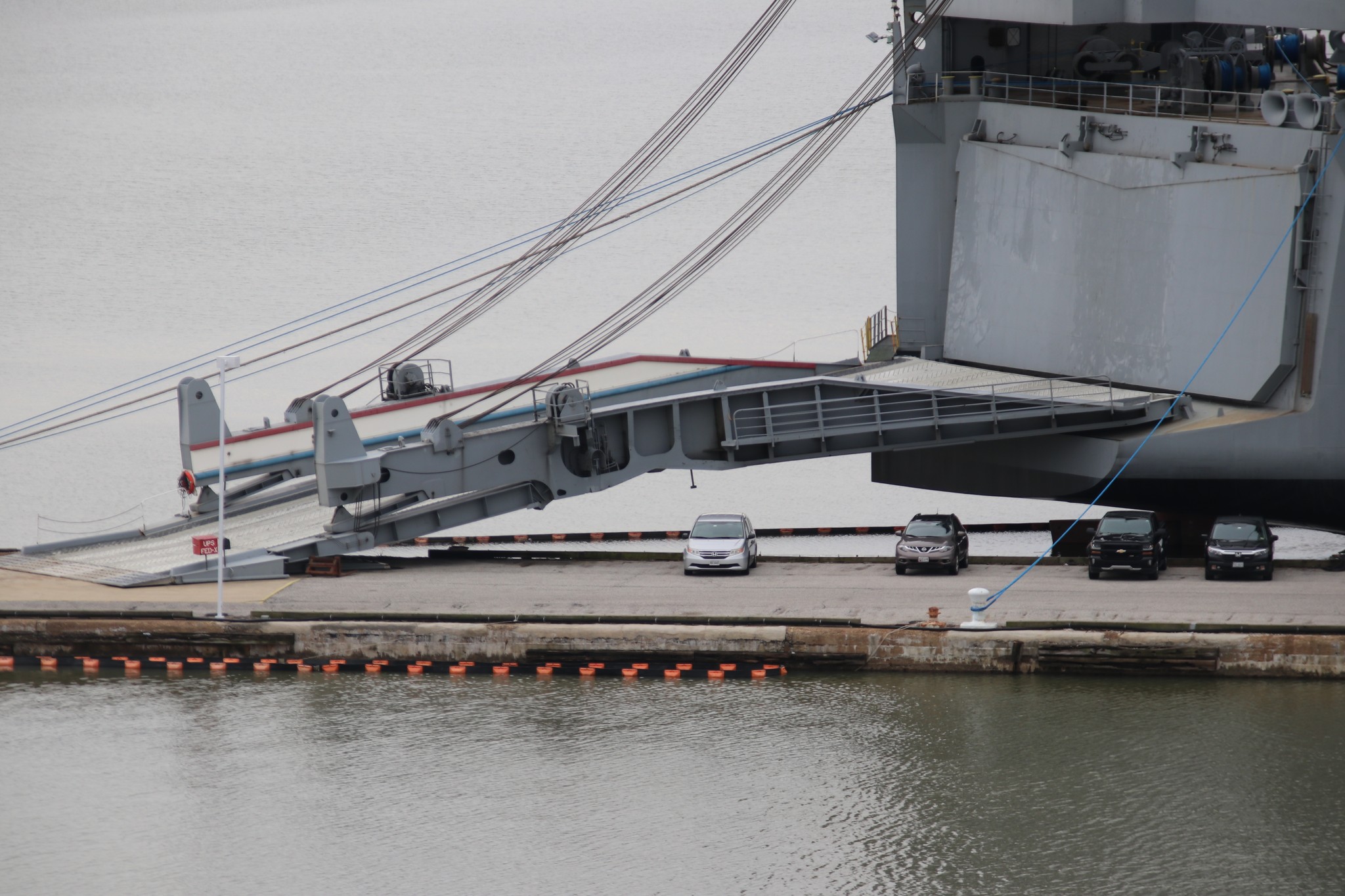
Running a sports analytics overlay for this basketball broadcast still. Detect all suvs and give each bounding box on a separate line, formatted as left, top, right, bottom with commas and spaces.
1201, 514, 1279, 581
1086, 510, 1168, 580
893, 511, 969, 574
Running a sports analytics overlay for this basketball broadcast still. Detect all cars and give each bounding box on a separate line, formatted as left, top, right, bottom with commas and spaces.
682, 512, 756, 576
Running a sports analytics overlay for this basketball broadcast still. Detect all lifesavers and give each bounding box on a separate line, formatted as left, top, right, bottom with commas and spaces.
181, 471, 194, 494
